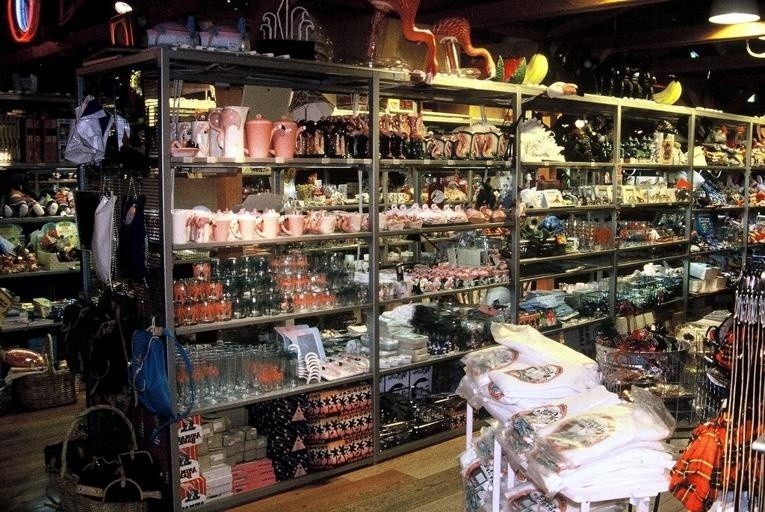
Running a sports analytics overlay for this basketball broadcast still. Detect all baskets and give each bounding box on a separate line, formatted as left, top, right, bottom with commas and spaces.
36, 249, 81, 272
44, 404, 163, 512
14, 333, 76, 410
0, 378, 13, 415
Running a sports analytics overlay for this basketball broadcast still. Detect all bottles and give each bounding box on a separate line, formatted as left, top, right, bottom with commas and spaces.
517, 306, 557, 332
59, 359, 69, 372
302, 114, 426, 159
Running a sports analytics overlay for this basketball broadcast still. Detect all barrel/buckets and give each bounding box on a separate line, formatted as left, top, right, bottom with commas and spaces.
676, 167, 706, 192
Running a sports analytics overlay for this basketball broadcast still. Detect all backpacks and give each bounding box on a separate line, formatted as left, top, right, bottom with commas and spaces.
54, 281, 195, 443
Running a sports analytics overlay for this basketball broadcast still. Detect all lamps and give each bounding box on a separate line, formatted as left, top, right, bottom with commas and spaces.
707, 0, 760, 25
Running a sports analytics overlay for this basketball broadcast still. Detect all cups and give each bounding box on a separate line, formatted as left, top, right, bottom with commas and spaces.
171, 201, 368, 246
175, 339, 299, 411
559, 217, 612, 253
169, 105, 250, 162
173, 238, 369, 328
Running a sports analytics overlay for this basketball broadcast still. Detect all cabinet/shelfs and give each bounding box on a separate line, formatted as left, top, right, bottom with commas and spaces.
0, 90, 76, 337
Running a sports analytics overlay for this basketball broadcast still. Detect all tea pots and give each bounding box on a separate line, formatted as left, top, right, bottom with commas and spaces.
244, 113, 305, 158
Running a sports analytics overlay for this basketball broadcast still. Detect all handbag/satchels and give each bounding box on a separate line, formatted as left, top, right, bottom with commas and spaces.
63, 71, 151, 293
